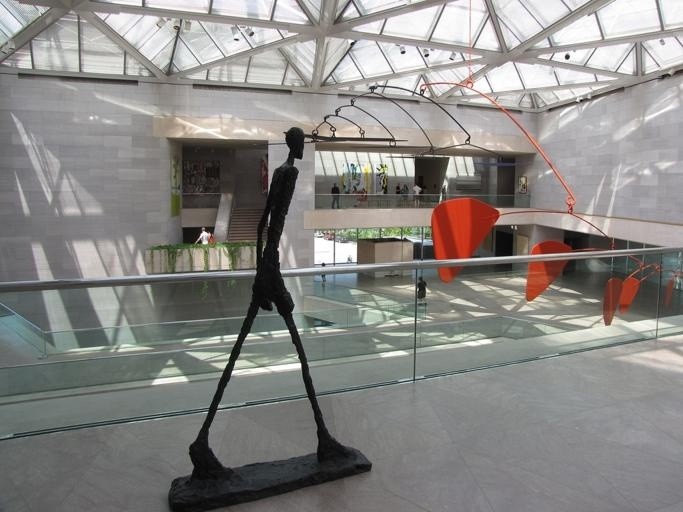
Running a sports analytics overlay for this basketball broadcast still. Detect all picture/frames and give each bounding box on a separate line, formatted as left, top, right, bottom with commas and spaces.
518, 176, 527, 194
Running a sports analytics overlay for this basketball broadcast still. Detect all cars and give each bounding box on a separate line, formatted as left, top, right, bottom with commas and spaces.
315, 232, 347, 243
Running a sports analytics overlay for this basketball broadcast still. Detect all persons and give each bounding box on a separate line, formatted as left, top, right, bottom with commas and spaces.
412, 183, 423, 208
330, 183, 340, 209
400, 184, 409, 208
206, 228, 216, 243
416, 277, 427, 306
193, 227, 208, 245
395, 182, 401, 194
